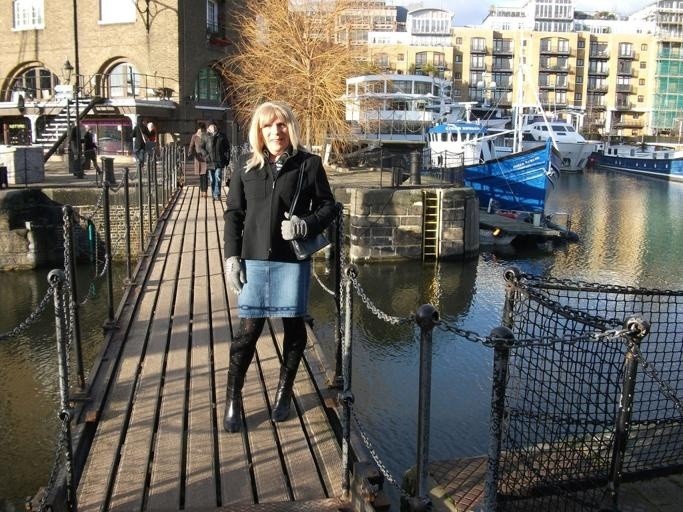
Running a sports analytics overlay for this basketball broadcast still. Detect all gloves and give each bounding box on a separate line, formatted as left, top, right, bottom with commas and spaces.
226, 256, 246, 294
281, 212, 307, 241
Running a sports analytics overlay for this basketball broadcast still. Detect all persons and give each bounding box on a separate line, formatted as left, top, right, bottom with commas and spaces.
83, 128, 103, 175
222, 99, 337, 434
129, 116, 158, 168
188, 118, 232, 201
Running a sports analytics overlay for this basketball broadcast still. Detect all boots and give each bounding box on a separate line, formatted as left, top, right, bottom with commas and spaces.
224, 372, 242, 433
270, 366, 297, 421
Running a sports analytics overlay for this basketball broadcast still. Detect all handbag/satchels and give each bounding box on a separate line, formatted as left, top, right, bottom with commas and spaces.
290, 214, 330, 260
145, 141, 155, 151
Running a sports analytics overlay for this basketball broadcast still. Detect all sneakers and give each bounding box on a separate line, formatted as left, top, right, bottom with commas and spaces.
200, 192, 220, 201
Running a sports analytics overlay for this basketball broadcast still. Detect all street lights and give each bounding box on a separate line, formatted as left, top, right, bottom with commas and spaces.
60, 56, 74, 174
